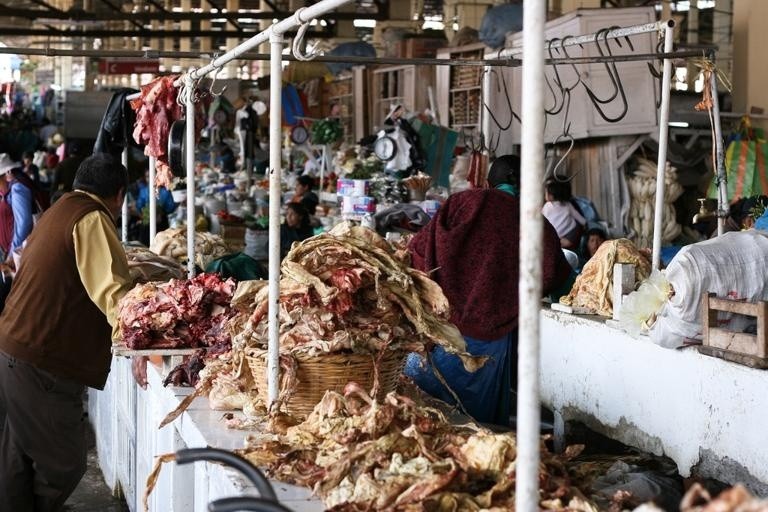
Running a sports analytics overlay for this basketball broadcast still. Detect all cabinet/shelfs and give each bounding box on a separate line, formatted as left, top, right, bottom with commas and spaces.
322, 76, 355, 141
372, 64, 429, 129
435, 42, 484, 129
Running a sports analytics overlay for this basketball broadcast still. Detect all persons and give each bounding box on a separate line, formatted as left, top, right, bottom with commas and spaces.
406, 153, 574, 421
744, 195, 766, 228
547, 175, 585, 253
294, 177, 318, 213
0, 91, 77, 280
580, 229, 607, 265
129, 172, 173, 239
545, 186, 586, 239
708, 200, 742, 239
2, 155, 133, 511
265, 204, 312, 256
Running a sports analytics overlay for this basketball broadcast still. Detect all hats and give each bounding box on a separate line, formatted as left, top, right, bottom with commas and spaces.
0, 153, 22, 176
